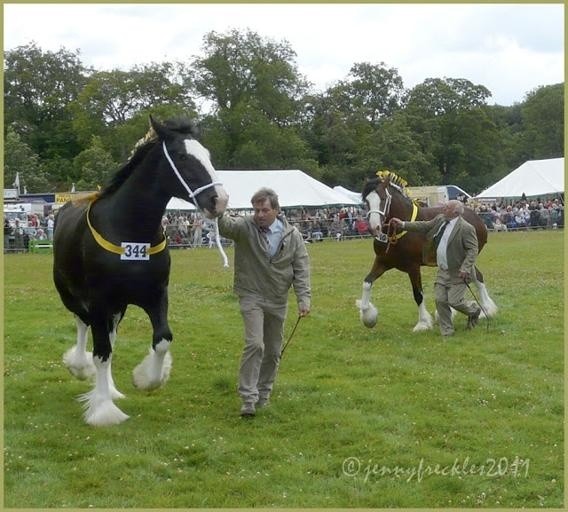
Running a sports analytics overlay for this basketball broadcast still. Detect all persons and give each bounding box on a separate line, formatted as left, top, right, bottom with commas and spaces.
3, 191, 565, 252
213, 185, 313, 419
387, 199, 482, 339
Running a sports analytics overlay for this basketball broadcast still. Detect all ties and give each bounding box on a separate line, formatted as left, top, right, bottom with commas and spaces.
434, 221, 449, 250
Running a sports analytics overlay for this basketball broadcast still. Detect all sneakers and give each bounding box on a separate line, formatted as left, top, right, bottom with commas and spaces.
468, 308, 480, 327
241, 403, 255, 415
256, 398, 269, 408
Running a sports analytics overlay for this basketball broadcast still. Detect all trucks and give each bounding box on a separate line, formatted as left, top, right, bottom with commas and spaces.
4, 209, 29, 237
403, 185, 481, 212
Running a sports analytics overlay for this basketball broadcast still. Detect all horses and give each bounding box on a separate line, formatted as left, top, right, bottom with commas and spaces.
353, 174, 499, 332
52, 111, 230, 431
